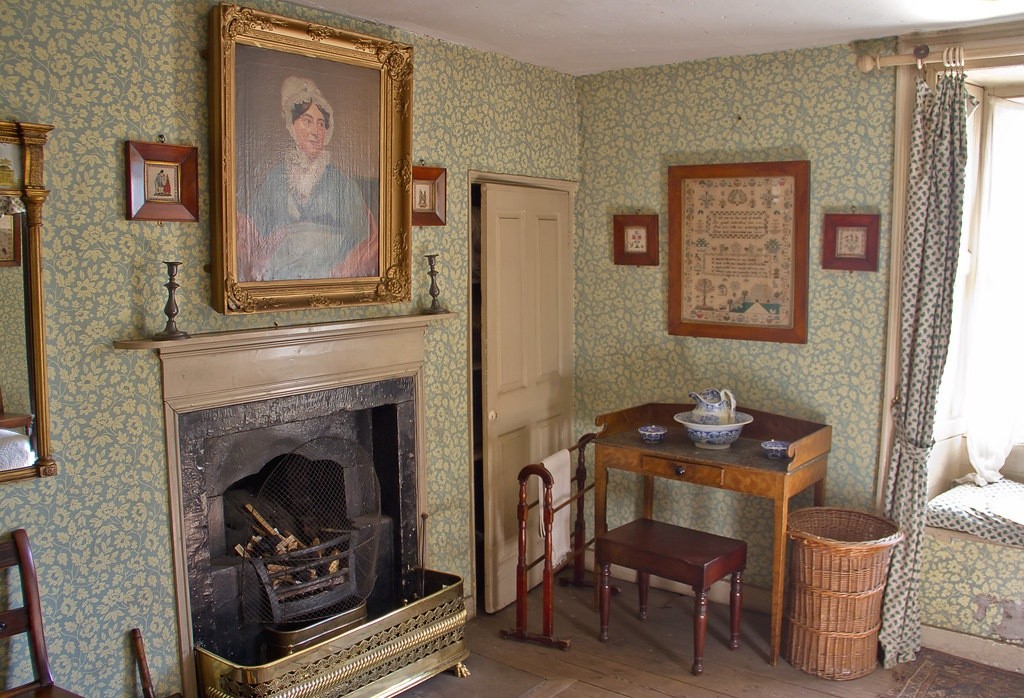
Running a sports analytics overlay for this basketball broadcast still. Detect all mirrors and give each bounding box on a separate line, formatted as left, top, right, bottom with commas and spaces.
2, 117, 57, 489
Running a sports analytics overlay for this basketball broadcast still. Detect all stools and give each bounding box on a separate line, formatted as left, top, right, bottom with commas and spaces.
592, 518, 747, 677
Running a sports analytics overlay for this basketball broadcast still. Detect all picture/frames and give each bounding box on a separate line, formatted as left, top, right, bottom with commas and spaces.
126, 139, 200, 223
213, 2, 414, 317
667, 159, 808, 345
411, 164, 446, 227
821, 213, 881, 272
612, 213, 660, 267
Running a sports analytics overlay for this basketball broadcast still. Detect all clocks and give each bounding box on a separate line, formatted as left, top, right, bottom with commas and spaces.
0, 158, 23, 269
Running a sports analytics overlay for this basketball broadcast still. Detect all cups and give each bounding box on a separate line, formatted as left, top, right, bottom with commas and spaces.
760, 439, 792, 459
638, 425, 668, 444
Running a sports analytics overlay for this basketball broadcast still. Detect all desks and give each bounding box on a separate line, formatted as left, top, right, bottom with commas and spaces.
589, 399, 834, 666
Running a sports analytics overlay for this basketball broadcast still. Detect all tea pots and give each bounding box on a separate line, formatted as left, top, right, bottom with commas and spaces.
687, 388, 737, 424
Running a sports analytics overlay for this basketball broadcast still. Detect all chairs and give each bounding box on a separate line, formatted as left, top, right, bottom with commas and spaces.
1, 524, 87, 698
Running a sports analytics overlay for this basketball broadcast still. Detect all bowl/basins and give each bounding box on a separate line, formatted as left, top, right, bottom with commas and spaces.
673, 409, 754, 449
